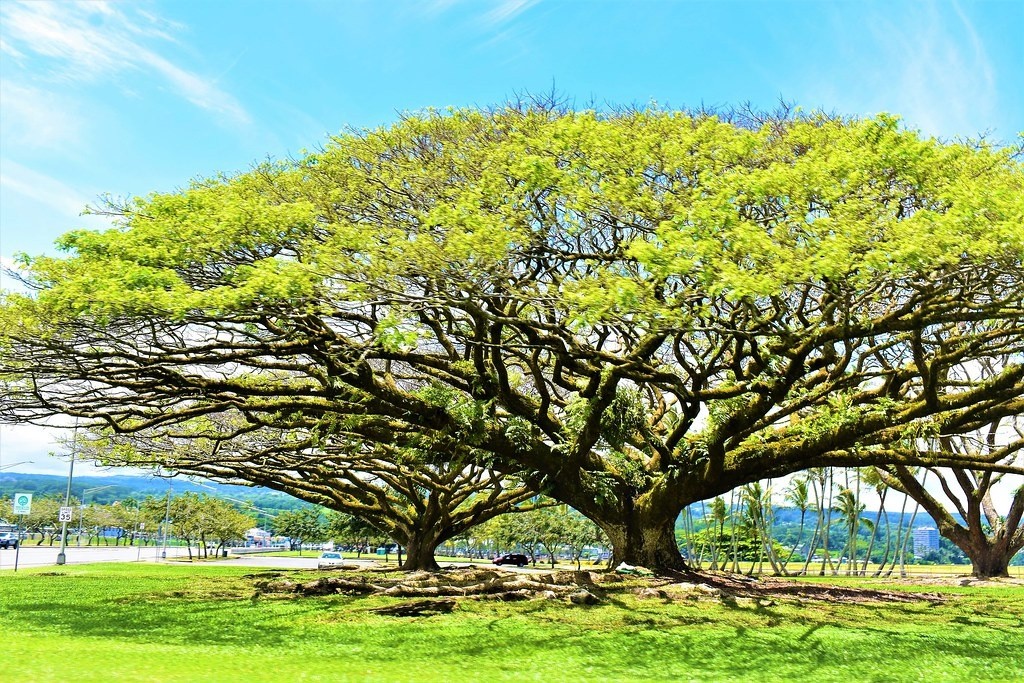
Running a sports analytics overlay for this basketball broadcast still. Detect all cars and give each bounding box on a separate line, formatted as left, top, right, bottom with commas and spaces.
55, 535, 71, 541
207, 542, 215, 548
0, 532, 18, 550
318, 552, 344, 568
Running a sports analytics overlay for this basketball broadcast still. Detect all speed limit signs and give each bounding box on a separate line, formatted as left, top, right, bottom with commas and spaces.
59, 507, 72, 522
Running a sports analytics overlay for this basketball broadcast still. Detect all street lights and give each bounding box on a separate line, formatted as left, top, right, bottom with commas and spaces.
77, 485, 117, 547
0, 460, 35, 470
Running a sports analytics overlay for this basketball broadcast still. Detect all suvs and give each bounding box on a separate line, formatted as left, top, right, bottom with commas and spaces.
492, 554, 528, 567
